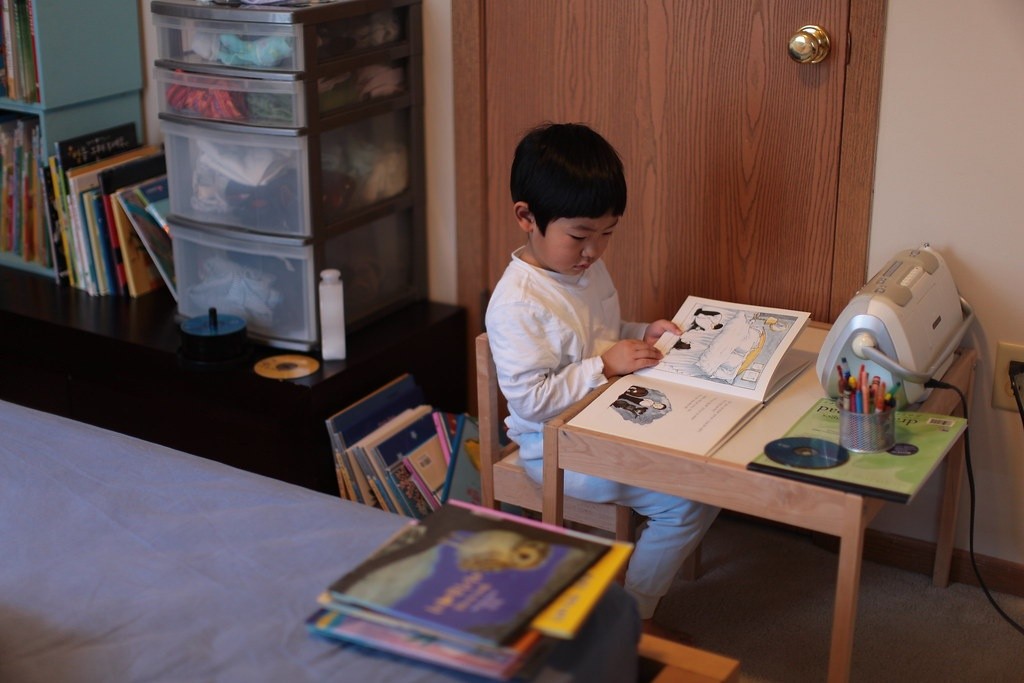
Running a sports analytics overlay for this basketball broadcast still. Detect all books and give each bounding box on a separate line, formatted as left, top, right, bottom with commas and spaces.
306, 372, 635, 683
0, 0, 41, 104
567, 295, 819, 506
0, 120, 177, 300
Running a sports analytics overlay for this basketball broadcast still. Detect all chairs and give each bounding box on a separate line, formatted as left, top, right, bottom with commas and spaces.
474, 333, 704, 581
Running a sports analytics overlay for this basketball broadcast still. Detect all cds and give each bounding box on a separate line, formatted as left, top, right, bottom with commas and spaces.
764, 436, 850, 469
253, 355, 321, 379
179, 315, 248, 360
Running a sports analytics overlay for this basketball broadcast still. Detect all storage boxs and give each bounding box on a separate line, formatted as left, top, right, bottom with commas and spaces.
148, 0, 426, 351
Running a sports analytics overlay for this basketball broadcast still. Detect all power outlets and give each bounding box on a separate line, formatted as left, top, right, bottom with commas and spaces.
990, 341, 1024, 414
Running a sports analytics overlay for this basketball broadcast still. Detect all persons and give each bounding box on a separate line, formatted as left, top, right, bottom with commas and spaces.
486, 123, 720, 624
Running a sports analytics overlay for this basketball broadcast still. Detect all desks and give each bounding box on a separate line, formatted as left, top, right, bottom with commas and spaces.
540, 317, 977, 682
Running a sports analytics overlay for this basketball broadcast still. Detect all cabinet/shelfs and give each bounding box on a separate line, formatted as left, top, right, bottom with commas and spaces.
0, 0, 145, 280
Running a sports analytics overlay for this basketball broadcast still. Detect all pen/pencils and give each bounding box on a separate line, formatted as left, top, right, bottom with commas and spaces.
834, 356, 903, 455
